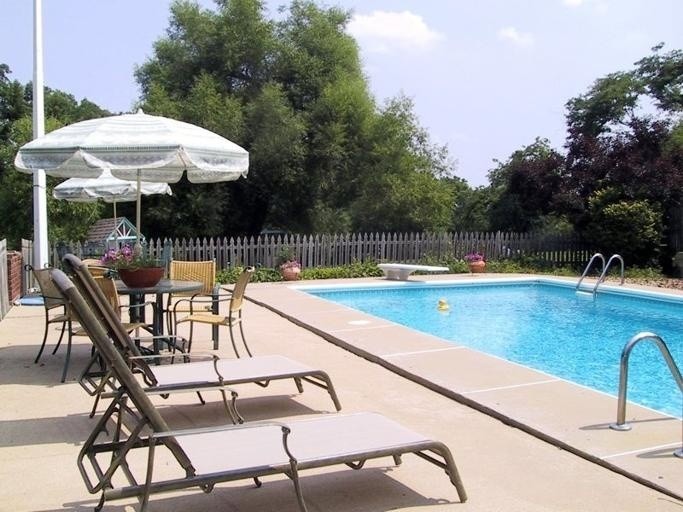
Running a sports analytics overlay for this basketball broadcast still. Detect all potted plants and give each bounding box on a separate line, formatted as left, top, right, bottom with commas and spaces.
276, 245, 299, 281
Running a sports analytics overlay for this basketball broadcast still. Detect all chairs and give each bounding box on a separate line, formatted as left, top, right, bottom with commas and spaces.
17, 256, 258, 383
45, 253, 467, 510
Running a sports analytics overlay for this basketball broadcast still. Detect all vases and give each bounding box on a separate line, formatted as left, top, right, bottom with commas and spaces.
468, 261, 485, 273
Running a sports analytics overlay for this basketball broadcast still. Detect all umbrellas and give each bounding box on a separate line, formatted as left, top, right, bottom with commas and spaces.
52, 170, 172, 254
14, 108, 249, 339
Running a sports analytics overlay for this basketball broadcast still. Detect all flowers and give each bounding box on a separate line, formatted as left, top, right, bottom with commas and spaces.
100, 245, 158, 270
466, 252, 484, 260
